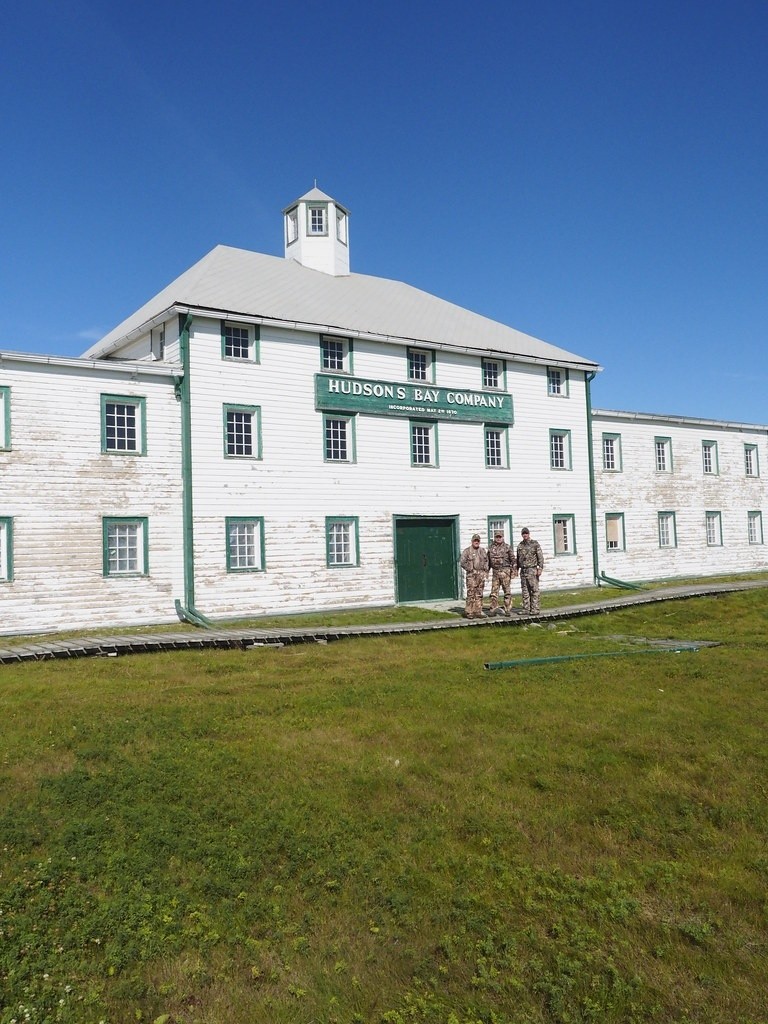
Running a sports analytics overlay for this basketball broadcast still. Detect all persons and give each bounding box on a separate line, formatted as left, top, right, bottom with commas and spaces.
515, 527, 543, 614
486, 531, 515, 617
461, 534, 489, 619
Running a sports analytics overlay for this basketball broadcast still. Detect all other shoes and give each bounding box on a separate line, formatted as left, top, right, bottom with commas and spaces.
467, 613, 474, 618
517, 609, 530, 615
488, 611, 496, 616
505, 611, 511, 617
530, 609, 539, 615
474, 612, 487, 618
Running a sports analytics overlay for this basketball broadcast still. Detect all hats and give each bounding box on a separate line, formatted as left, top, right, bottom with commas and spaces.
472, 534, 480, 541
521, 527, 529, 533
494, 532, 503, 537
